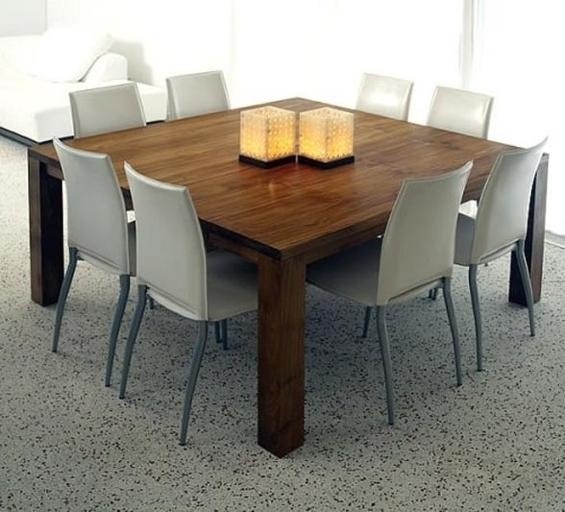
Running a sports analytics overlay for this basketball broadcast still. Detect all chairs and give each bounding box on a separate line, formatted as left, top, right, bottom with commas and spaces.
69, 85, 144, 142
353, 72, 414, 122
117, 160, 262, 445
309, 158, 475, 426
167, 72, 229, 124
423, 85, 493, 299
431, 149, 543, 375
53, 132, 222, 389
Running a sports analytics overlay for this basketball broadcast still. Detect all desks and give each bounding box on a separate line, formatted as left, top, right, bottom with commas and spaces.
28, 98, 548, 458
